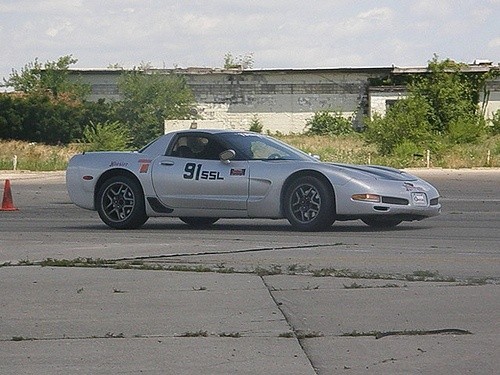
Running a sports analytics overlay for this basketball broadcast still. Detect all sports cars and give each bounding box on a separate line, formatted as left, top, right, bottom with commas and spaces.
65, 128, 443, 232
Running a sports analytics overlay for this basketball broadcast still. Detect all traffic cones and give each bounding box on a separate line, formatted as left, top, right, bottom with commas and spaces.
0, 178, 19, 211
